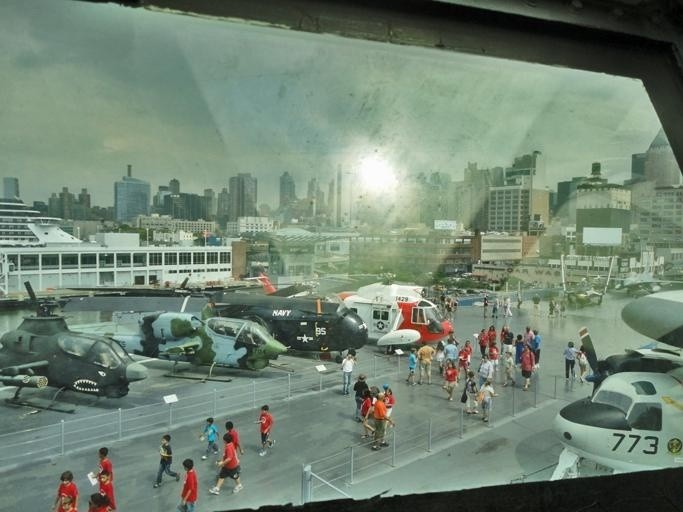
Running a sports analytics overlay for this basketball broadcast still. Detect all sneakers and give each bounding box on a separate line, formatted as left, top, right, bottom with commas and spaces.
355, 417, 390, 450
565, 374, 585, 383
201, 448, 220, 461
406, 378, 432, 386
466, 408, 488, 423
344, 393, 351, 397
232, 483, 243, 494
153, 473, 181, 486
503, 381, 531, 391
442, 384, 454, 400
259, 439, 276, 456
484, 315, 512, 318
208, 486, 223, 496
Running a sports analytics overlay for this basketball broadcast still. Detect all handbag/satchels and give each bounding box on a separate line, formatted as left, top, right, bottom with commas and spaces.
460, 392, 467, 402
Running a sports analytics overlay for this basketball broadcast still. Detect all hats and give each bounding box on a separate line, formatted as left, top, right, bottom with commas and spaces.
356, 374, 367, 381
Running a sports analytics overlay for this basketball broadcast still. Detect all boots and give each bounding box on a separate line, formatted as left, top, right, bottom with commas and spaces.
439, 366, 443, 374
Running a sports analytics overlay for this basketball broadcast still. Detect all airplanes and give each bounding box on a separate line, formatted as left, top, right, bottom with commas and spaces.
553, 293, 682, 473
600, 263, 682, 296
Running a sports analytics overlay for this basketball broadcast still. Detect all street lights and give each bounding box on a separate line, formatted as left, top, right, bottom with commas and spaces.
118, 227, 122, 233
204, 229, 207, 247
76, 226, 81, 239
145, 227, 149, 247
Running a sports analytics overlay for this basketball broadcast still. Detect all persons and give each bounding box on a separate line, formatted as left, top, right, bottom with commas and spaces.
200, 418, 220, 460
177, 459, 197, 512
51, 447, 116, 512
202, 302, 216, 320
341, 281, 588, 452
153, 435, 181, 487
254, 405, 275, 455
225, 421, 244, 456
208, 433, 243, 494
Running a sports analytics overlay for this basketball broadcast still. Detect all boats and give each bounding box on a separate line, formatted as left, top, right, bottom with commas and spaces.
0, 188, 83, 248
